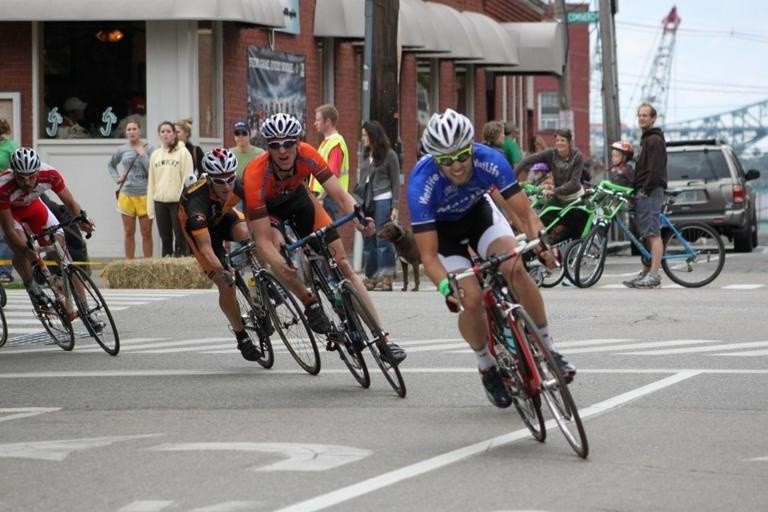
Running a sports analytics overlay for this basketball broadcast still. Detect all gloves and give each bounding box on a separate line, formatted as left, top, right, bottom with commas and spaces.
439, 278, 466, 313
532, 242, 561, 268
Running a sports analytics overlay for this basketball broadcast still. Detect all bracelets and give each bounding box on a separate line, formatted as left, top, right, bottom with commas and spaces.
22, 245, 29, 256
437, 278, 452, 296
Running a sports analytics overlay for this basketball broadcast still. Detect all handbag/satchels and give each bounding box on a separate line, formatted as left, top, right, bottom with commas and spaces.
353, 181, 376, 218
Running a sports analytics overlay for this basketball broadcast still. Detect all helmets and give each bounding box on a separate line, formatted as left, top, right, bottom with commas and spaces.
259, 113, 303, 139
202, 148, 239, 174
610, 141, 634, 156
530, 163, 549, 171
421, 108, 475, 155
11, 146, 41, 176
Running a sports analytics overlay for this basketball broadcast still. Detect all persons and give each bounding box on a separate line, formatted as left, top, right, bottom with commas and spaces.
0, 117, 22, 283
242, 111, 406, 365
179, 149, 300, 362
0, 147, 107, 339
404, 102, 577, 410
110, 96, 147, 138
483, 120, 589, 235
111, 117, 265, 261
609, 142, 636, 192
356, 120, 400, 291
248, 102, 298, 130
307, 103, 350, 222
53, 97, 107, 139
621, 101, 668, 290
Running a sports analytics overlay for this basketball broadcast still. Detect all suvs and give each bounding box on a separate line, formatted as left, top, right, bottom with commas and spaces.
661, 140, 759, 252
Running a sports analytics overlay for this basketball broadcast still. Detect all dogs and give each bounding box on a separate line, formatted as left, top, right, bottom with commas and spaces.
377, 220, 425, 292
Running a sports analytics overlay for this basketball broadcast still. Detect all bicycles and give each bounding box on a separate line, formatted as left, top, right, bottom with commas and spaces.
573, 185, 724, 286
280, 204, 407, 401
0, 282, 11, 348
18, 210, 119, 357
513, 181, 602, 287
440, 232, 591, 460
223, 239, 322, 377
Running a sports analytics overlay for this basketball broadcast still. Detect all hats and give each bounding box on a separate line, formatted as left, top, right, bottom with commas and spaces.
233, 122, 251, 132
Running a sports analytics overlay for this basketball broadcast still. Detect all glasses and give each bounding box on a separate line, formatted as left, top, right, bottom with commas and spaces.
234, 131, 247, 136
14, 172, 39, 181
435, 144, 472, 165
267, 140, 295, 148
207, 174, 237, 185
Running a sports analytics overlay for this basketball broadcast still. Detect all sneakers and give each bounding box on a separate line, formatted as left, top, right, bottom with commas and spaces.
255, 283, 286, 297
546, 351, 574, 380
83, 311, 106, 334
25, 280, 48, 306
304, 300, 330, 333
478, 365, 510, 407
378, 341, 406, 362
237, 335, 260, 361
622, 271, 645, 287
634, 272, 662, 288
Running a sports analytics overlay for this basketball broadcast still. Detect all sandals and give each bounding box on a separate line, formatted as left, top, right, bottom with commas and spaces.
373, 281, 392, 291
365, 278, 379, 289
